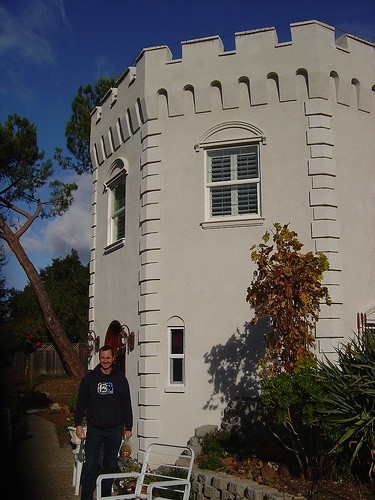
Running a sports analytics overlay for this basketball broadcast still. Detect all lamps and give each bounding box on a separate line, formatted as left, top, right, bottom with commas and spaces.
86, 330, 99, 353
118, 324, 134, 353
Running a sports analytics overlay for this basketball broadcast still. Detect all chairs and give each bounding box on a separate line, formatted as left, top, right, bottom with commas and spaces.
71, 437, 123, 495
96, 442, 194, 500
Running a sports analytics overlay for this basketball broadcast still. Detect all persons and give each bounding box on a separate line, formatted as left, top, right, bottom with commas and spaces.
74, 345, 133, 500
23, 332, 41, 375
